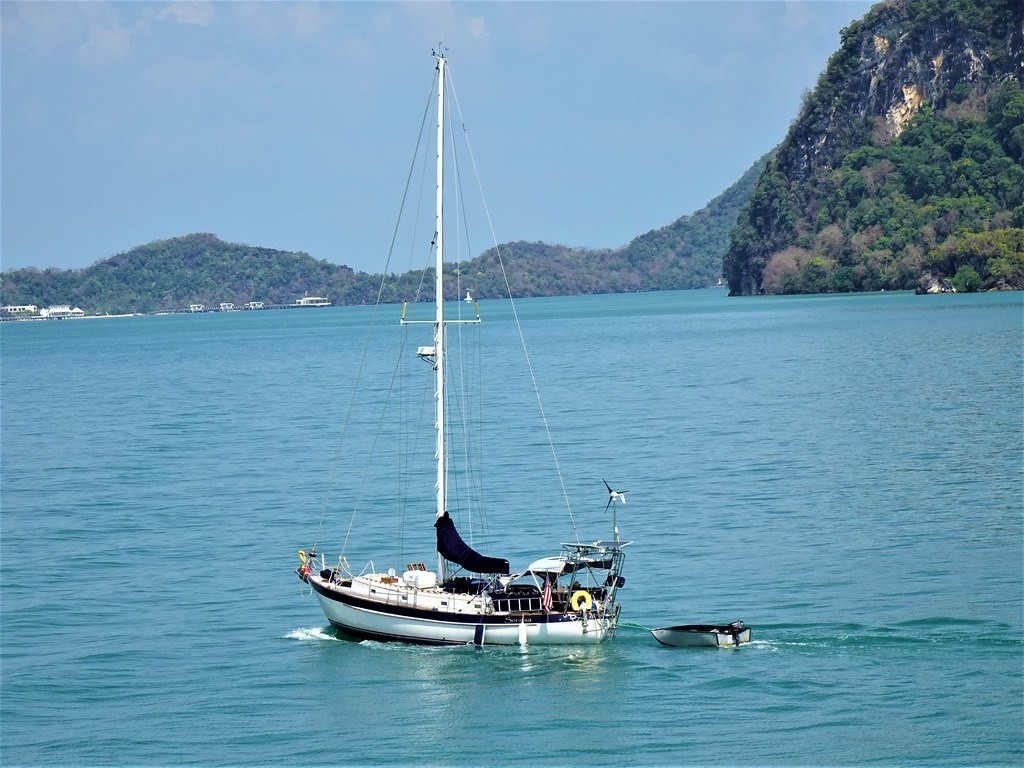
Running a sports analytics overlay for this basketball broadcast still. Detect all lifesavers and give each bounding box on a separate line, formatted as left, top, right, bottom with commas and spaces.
571, 590, 592, 612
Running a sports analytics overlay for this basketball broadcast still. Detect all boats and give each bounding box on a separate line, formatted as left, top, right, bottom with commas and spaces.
650, 622, 751, 649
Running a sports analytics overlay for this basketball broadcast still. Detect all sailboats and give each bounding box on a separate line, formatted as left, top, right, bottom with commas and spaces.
287, 38, 637, 651
